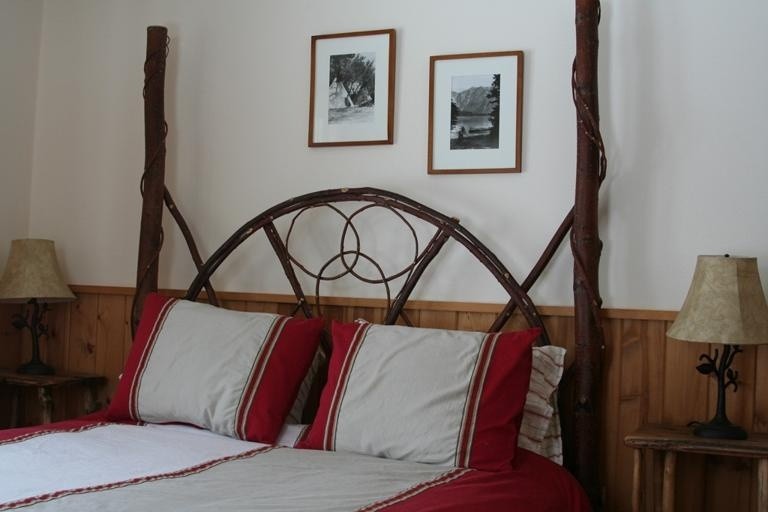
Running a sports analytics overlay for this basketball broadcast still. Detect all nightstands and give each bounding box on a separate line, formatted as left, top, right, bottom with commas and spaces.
623, 423, 768, 512
0, 370, 106, 431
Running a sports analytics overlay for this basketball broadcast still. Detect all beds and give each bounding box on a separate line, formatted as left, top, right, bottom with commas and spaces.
0, 0, 608, 512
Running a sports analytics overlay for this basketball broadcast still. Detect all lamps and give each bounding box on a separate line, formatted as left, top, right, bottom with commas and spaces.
0, 239, 77, 375
666, 254, 768, 441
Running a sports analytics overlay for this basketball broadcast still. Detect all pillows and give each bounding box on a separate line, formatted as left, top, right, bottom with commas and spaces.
518, 343, 567, 466
106, 294, 332, 445
296, 320, 542, 475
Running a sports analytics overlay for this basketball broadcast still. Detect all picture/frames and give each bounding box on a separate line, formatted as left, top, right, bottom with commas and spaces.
308, 28, 396, 147
427, 50, 524, 175
